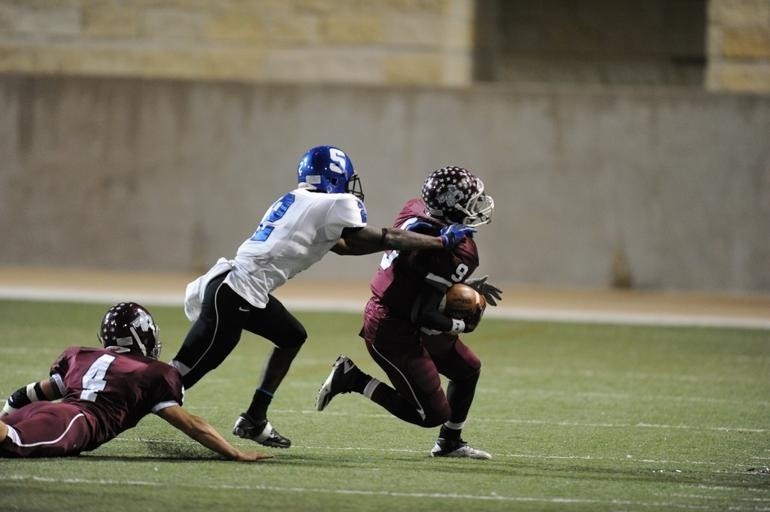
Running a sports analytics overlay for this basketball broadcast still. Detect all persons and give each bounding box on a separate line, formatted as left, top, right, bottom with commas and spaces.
166, 146, 476, 448
315, 167, 502, 459
0, 301, 274, 462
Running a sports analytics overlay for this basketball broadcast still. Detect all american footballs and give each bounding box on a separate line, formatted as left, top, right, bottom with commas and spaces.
446, 282, 485, 323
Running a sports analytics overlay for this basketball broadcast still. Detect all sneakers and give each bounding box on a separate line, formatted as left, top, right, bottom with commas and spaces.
430, 442, 491, 460
232, 412, 292, 448
316, 356, 359, 411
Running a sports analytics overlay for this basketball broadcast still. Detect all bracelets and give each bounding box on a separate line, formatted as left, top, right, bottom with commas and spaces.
444, 318, 466, 335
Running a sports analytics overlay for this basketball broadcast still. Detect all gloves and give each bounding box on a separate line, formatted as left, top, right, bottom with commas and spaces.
466, 275, 503, 306
440, 222, 477, 247
463, 303, 488, 332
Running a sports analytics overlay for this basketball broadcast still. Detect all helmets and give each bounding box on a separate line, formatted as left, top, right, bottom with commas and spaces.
300, 148, 364, 201
423, 167, 495, 228
101, 302, 160, 360
